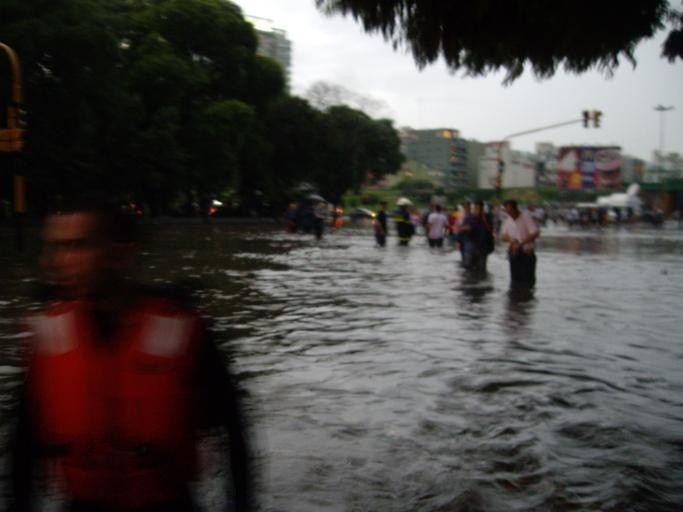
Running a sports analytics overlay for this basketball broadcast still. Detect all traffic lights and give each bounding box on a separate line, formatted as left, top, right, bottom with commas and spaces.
592, 107, 603, 129
16, 108, 28, 128
580, 108, 588, 129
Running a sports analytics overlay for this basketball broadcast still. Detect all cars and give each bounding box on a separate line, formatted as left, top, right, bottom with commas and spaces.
117, 193, 380, 220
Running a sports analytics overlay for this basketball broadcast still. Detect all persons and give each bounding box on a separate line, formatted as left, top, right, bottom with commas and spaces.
126, 201, 143, 226
290, 189, 343, 239
499, 199, 540, 292
0, 195, 260, 512
373, 196, 494, 273
526, 204, 662, 228
